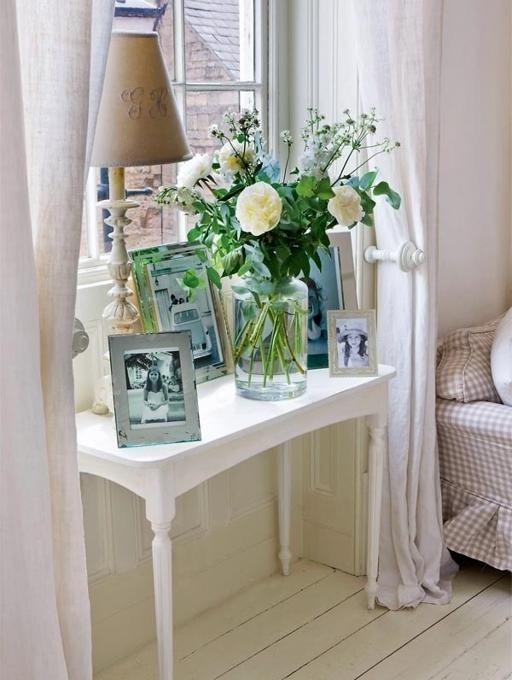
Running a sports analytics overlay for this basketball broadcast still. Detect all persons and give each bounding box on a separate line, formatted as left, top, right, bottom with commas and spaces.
141, 366, 176, 423
169, 294, 193, 320
337, 322, 369, 369
299, 277, 327, 342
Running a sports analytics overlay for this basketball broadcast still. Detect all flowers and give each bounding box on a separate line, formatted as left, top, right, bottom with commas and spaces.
154, 102, 402, 393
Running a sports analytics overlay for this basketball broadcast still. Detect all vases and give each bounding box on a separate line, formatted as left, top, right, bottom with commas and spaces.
228, 270, 312, 404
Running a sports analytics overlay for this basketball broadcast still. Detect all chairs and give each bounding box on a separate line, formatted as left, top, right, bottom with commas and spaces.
427, 306, 512, 580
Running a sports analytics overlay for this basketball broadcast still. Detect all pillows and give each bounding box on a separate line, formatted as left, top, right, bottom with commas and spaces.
487, 300, 512, 407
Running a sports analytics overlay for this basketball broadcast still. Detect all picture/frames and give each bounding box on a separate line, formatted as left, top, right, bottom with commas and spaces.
127, 237, 236, 387
286, 225, 364, 372
104, 330, 207, 451
325, 308, 382, 379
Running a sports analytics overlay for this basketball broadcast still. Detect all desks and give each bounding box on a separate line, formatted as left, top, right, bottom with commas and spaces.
67, 362, 404, 680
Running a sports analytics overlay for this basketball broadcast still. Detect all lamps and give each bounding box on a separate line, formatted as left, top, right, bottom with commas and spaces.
87, 28, 200, 417
